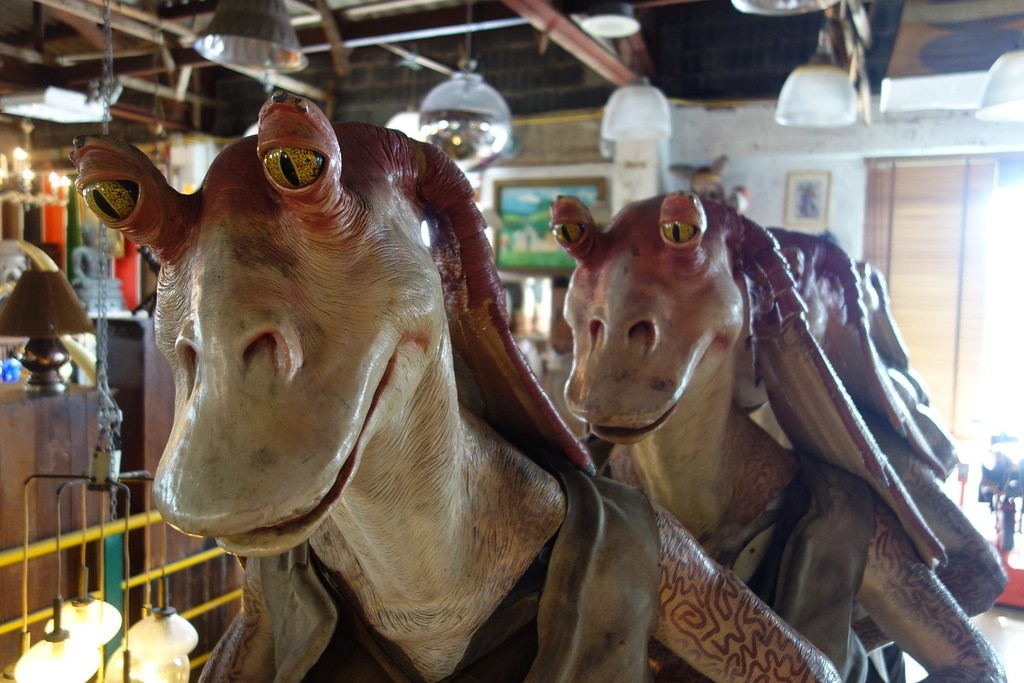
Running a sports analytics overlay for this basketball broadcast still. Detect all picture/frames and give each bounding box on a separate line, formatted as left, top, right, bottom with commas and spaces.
495, 178, 606, 271
784, 169, 831, 230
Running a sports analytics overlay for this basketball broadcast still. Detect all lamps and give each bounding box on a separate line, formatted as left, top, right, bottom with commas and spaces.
773, 14, 858, 125
195, 0, 309, 73
581, 0, 639, 39
0, 268, 97, 396
600, 37, 672, 141
974, 48, 1023, 122
0, 85, 114, 122
0, 1, 198, 683
419, 1, 512, 169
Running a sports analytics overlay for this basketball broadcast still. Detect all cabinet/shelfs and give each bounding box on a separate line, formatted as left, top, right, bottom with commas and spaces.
1, 333, 248, 677
